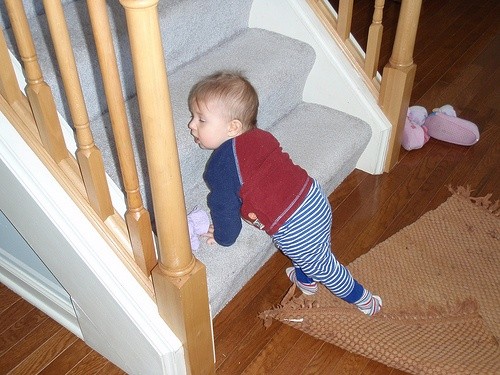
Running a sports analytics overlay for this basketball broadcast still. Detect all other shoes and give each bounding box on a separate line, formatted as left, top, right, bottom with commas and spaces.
427, 105, 480, 145
400, 105, 430, 150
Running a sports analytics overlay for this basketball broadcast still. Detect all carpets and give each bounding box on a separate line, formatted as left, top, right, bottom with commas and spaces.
260, 182, 499, 375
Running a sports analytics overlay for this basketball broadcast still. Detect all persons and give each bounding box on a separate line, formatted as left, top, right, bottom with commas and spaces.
188, 69, 383, 316
